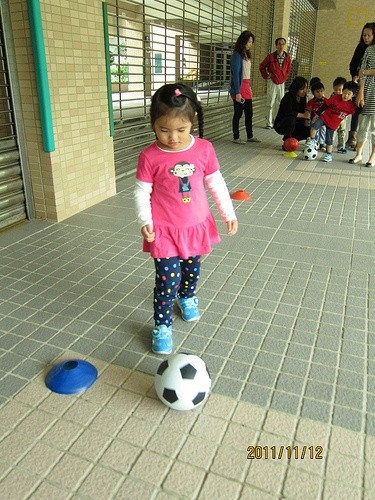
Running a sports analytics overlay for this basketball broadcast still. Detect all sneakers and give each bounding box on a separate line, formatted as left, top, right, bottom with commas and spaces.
305, 137, 317, 147
151, 319, 173, 355
176, 292, 200, 322
337, 147, 347, 153
321, 153, 333, 162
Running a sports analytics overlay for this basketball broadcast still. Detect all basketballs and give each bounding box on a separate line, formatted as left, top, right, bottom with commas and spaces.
284, 138, 298, 152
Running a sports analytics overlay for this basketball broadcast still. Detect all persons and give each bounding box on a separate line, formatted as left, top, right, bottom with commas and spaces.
231, 30, 260, 143
260, 21, 375, 168
135, 83, 239, 355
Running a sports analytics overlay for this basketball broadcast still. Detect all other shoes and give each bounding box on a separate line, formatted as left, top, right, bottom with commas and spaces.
232, 138, 247, 144
348, 154, 363, 164
365, 162, 375, 167
265, 122, 272, 129
247, 137, 262, 142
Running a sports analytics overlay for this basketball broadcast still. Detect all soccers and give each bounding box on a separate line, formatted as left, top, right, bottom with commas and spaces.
302, 147, 318, 160
155, 353, 213, 412
